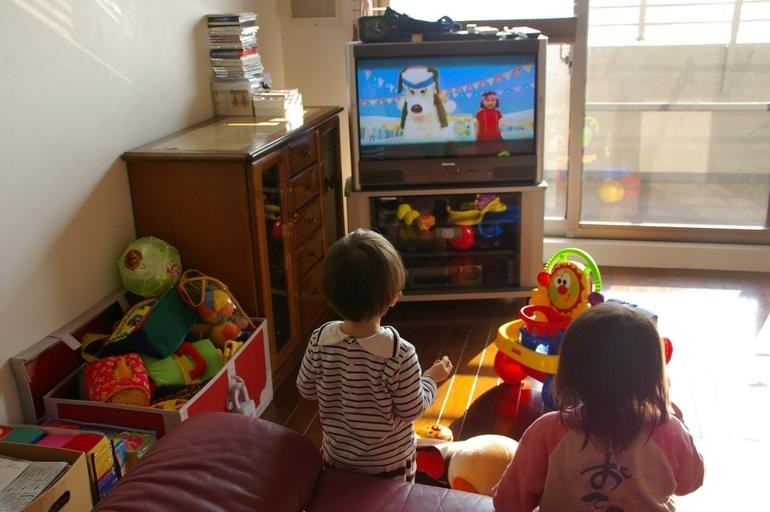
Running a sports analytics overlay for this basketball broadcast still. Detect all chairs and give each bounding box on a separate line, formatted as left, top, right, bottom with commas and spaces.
92, 412, 497, 512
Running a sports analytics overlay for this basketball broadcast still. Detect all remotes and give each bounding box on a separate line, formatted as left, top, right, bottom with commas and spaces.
512, 26, 541, 39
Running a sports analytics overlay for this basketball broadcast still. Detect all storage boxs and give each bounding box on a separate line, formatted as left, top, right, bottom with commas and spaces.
9, 290, 274, 442
1, 414, 155, 512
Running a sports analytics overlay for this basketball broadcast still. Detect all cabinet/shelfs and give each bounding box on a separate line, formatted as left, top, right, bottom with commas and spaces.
344, 180, 549, 310
119, 103, 349, 403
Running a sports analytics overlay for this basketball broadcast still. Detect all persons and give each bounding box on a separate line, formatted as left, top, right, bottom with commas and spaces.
493, 301, 705, 512
295, 228, 454, 483
475, 91, 503, 141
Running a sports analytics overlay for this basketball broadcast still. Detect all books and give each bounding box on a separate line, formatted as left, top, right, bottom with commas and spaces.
208, 11, 303, 118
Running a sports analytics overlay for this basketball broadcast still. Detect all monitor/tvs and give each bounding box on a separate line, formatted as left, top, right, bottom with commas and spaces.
345, 34, 548, 192
